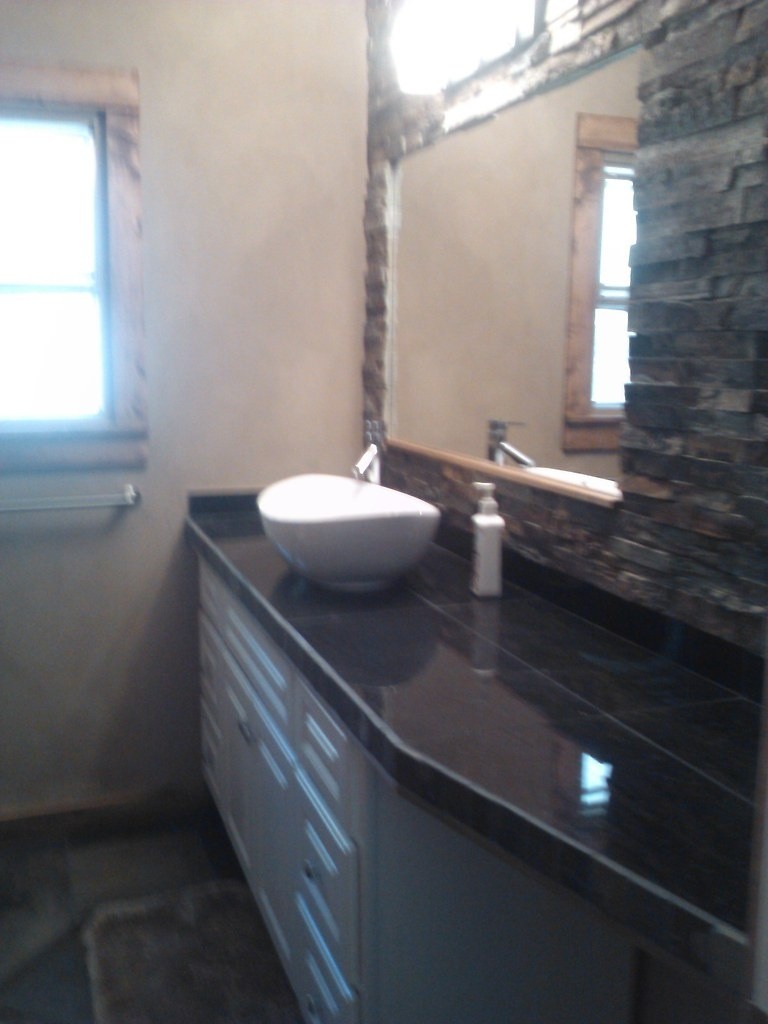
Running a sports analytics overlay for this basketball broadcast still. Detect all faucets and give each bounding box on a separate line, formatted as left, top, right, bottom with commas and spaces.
350, 417, 386, 486
483, 417, 540, 472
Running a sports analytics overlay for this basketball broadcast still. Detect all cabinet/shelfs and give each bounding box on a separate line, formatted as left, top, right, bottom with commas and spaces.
190, 552, 657, 1024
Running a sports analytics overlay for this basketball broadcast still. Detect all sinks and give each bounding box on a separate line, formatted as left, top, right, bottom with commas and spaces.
255, 470, 441, 595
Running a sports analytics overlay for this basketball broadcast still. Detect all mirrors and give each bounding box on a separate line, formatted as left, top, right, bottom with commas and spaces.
387, 45, 644, 499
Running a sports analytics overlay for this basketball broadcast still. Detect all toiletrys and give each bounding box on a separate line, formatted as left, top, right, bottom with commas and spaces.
468, 480, 506, 598
